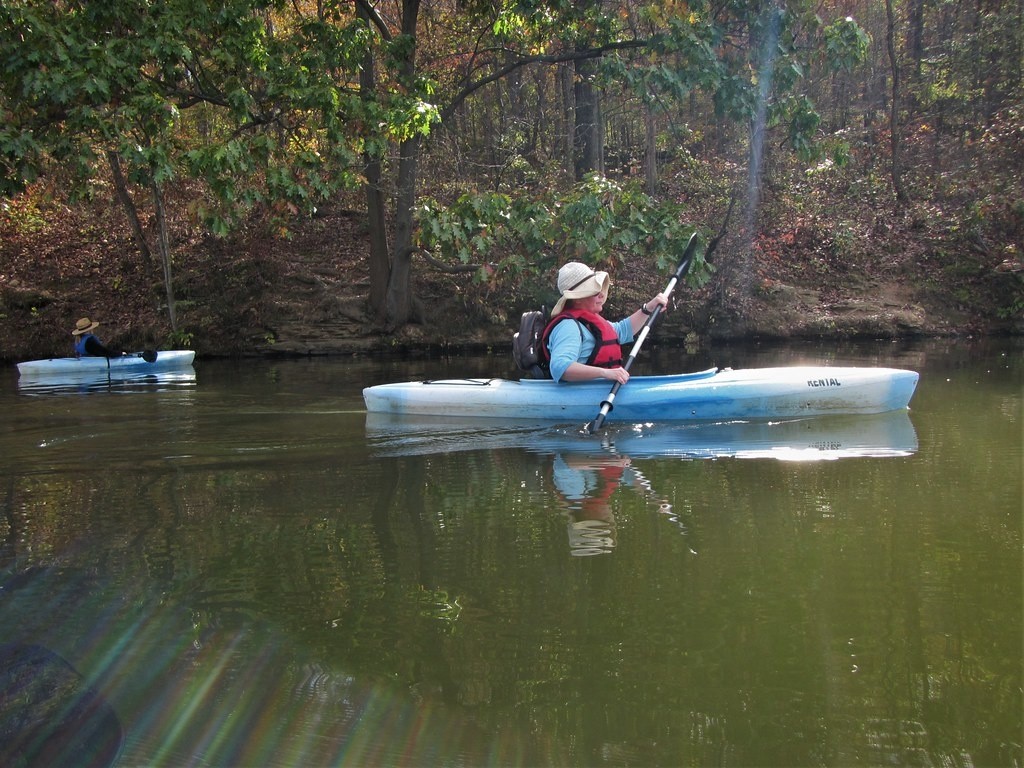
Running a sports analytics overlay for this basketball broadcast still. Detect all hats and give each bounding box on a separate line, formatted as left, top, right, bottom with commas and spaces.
72, 318, 99, 335
551, 262, 610, 316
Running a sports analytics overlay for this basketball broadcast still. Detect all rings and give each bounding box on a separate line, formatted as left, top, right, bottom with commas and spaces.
663, 298, 664, 299
622, 375, 625, 378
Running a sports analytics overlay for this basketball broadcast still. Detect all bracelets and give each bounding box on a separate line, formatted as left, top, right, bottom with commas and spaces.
642, 303, 652, 315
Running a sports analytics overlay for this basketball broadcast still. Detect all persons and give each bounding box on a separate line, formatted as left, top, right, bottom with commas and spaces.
546, 262, 667, 385
73, 318, 127, 356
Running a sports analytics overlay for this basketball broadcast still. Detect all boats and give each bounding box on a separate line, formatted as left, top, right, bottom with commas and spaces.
362, 367, 919, 421
16, 350, 196, 375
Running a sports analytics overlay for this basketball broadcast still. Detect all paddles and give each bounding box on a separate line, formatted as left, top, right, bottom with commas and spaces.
589, 232, 697, 435
124, 349, 158, 363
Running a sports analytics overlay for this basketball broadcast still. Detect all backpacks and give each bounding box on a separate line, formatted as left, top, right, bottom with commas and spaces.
512, 305, 602, 380
